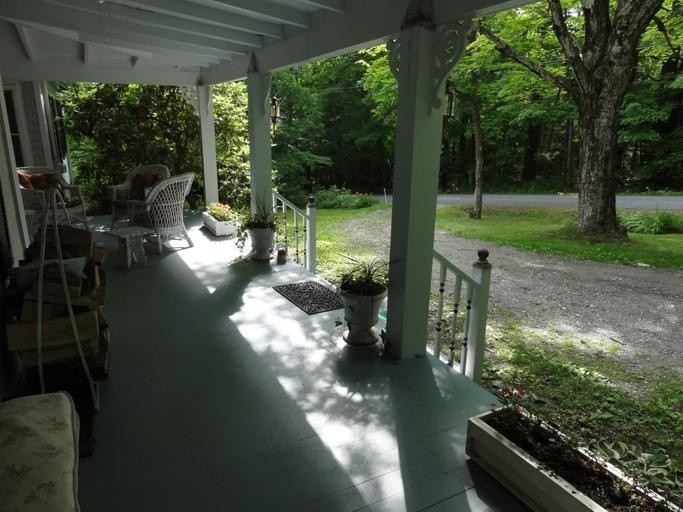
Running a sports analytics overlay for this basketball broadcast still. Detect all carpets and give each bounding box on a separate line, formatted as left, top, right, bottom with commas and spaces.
272, 281, 344, 314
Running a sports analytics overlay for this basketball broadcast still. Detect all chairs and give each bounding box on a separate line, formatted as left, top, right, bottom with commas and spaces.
16, 164, 194, 257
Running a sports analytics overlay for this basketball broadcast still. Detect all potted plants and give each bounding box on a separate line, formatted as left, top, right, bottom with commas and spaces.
235, 182, 279, 260
328, 257, 400, 346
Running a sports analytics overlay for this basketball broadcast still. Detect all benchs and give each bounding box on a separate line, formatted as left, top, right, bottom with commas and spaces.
0, 392, 81, 511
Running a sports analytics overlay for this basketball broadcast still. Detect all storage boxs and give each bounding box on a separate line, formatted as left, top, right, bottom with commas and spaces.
0, 224, 113, 377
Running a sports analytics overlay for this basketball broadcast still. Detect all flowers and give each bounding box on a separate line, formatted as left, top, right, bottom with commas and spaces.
206, 203, 235, 221
489, 371, 683, 512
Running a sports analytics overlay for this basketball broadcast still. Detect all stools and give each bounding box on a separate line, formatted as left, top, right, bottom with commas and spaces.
104, 226, 155, 271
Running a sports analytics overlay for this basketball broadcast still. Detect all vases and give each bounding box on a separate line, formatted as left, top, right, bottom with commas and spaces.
465, 404, 683, 512
203, 211, 236, 236
